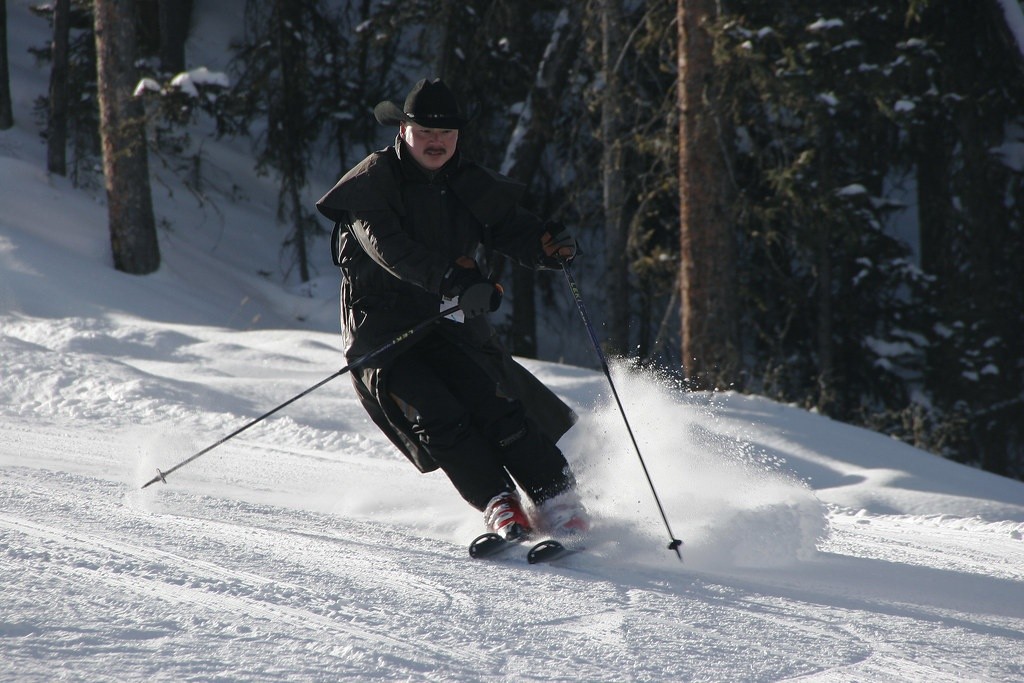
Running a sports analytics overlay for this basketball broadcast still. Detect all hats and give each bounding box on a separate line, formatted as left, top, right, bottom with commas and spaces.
373, 77, 497, 129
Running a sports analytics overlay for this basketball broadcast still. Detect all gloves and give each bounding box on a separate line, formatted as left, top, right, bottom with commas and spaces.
451, 268, 501, 319
539, 224, 577, 265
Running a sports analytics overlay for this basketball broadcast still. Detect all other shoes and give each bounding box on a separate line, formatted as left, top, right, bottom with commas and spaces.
489, 495, 533, 543
545, 500, 585, 536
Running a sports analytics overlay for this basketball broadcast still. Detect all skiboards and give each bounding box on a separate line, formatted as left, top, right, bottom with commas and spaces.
468, 518, 539, 560
526, 521, 634, 566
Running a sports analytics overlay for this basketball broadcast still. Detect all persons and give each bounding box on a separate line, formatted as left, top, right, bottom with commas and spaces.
315, 75, 594, 544
